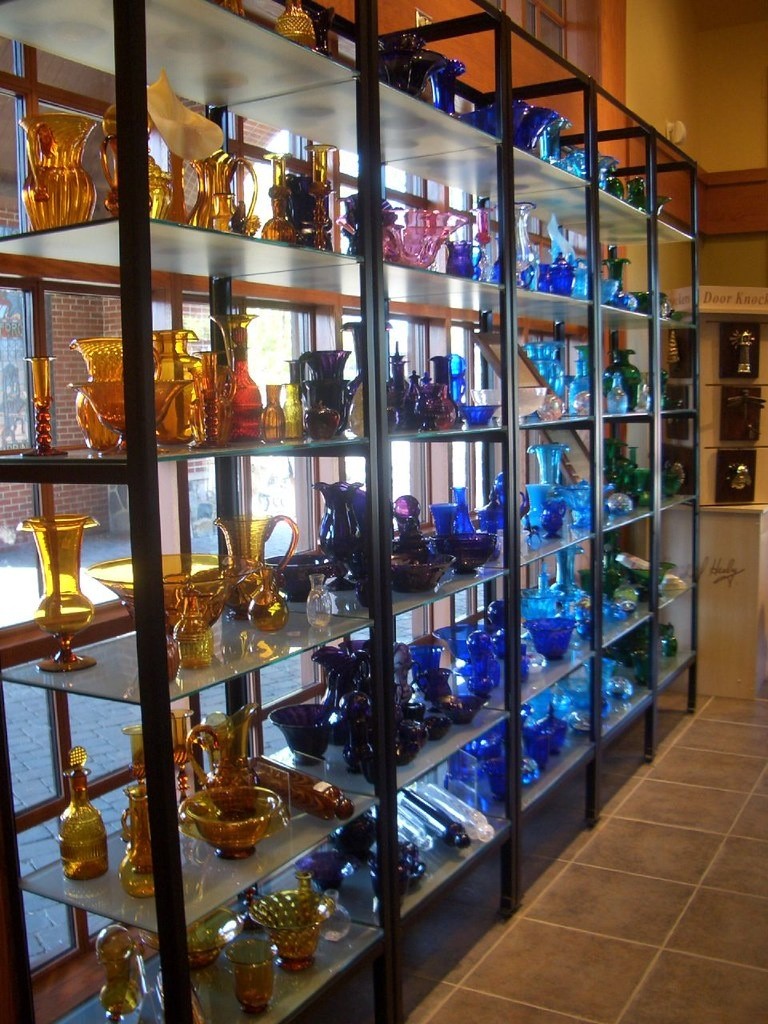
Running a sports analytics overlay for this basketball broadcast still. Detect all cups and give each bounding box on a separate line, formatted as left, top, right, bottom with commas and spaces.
226, 938, 278, 1012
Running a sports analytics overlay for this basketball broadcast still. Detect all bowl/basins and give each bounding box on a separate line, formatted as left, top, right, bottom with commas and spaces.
438, 695, 484, 723
577, 569, 622, 596
628, 562, 676, 585
371, 863, 425, 887
249, 889, 334, 968
85, 556, 261, 636
182, 789, 279, 857
457, 102, 559, 150
461, 406, 499, 426
140, 907, 242, 968
336, 210, 470, 269
600, 279, 619, 304
523, 617, 577, 659
429, 535, 497, 573
472, 386, 548, 425
65, 380, 192, 454
264, 555, 336, 599
268, 704, 344, 762
431, 624, 503, 675
391, 554, 456, 590
295, 851, 359, 890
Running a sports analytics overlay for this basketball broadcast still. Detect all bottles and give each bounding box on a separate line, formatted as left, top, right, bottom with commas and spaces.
284, 383, 303, 439
320, 890, 351, 941
402, 370, 425, 429
262, 385, 285, 443
59, 746, 108, 881
434, 385, 456, 429
420, 373, 432, 400
568, 350, 590, 416
606, 372, 627, 415
396, 806, 433, 852
422, 784, 494, 844
397, 788, 471, 848
386, 341, 410, 410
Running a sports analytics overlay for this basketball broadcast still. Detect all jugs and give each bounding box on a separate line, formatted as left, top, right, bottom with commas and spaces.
16, 0, 686, 1024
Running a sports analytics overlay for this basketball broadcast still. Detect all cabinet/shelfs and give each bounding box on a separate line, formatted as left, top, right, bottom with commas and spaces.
365, 0, 705, 1021
0, 0, 399, 1024
699, 310, 768, 506
